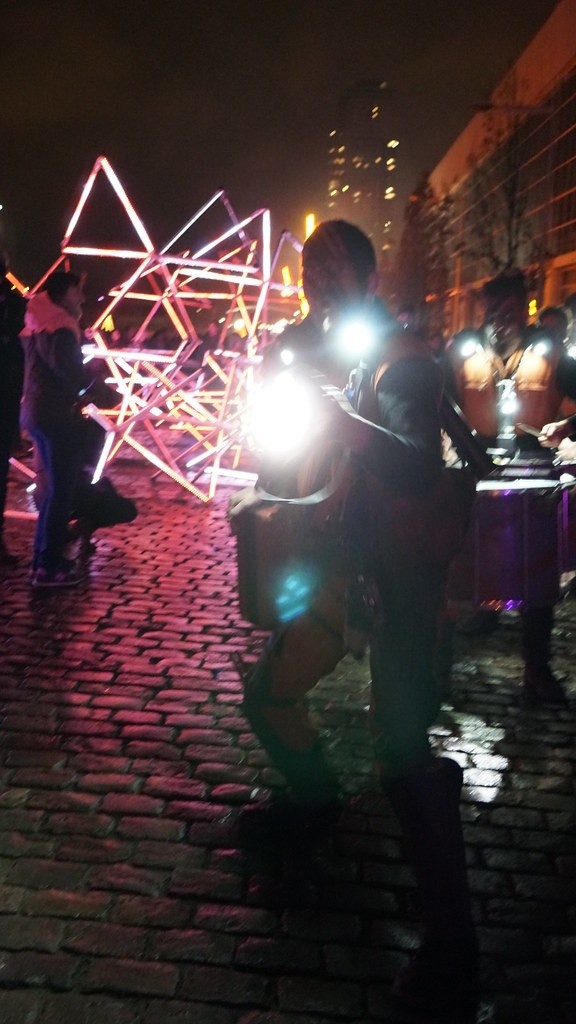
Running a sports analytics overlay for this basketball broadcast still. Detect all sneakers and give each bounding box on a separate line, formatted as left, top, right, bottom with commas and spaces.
523, 665, 566, 704
28, 561, 93, 587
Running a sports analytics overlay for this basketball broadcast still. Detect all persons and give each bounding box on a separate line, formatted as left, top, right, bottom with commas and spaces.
0, 250, 139, 583
212, 218, 576, 956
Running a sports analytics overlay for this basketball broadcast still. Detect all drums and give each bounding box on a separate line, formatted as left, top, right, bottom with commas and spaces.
444, 454, 575, 611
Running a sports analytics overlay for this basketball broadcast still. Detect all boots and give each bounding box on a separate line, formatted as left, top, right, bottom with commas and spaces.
388, 757, 481, 1024
239, 630, 344, 825
